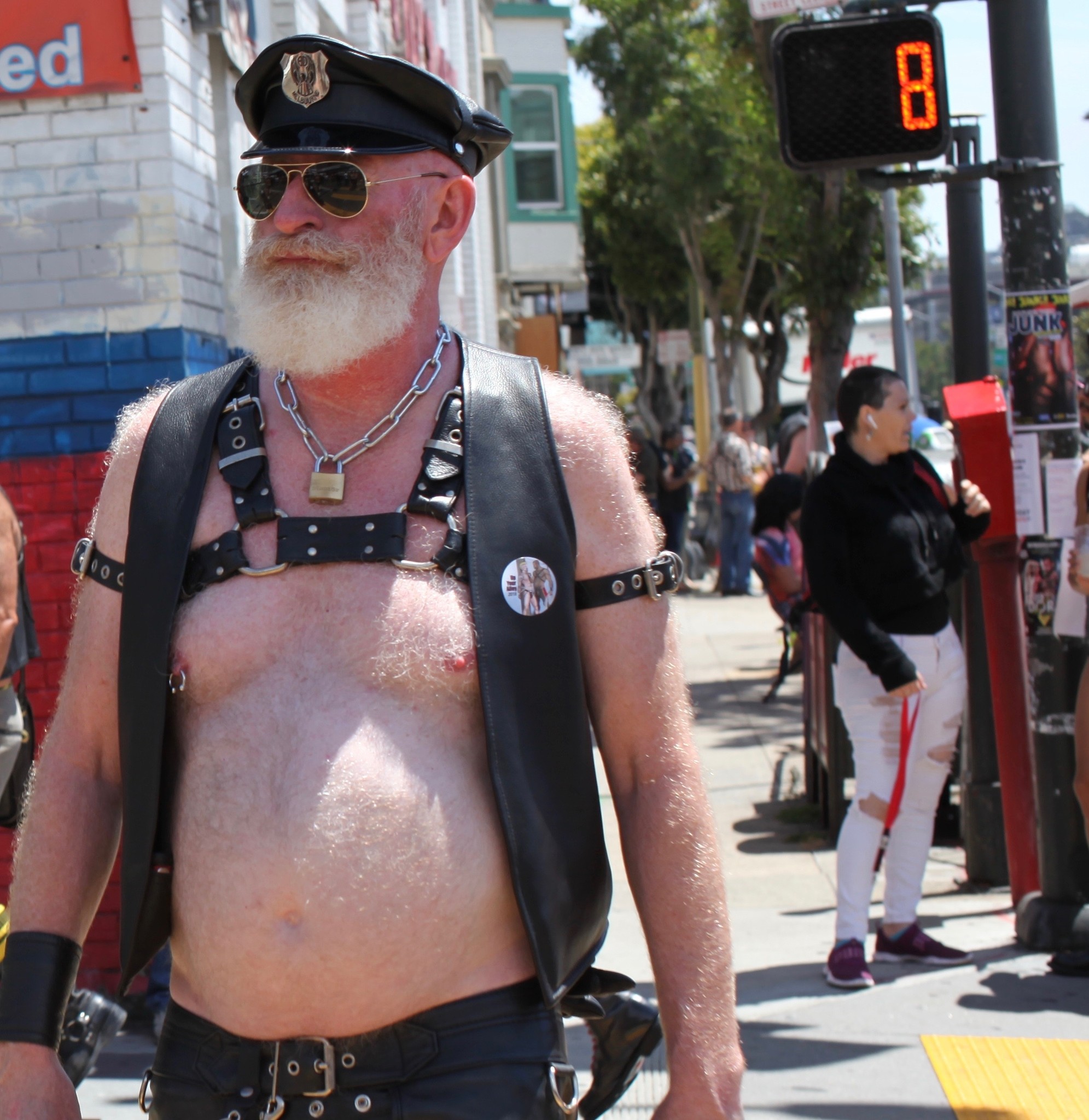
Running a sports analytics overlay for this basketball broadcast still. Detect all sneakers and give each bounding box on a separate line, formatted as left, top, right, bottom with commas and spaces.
872, 924, 976, 965
822, 939, 874, 988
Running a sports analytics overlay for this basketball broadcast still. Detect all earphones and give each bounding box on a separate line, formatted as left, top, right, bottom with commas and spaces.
866, 413, 878, 431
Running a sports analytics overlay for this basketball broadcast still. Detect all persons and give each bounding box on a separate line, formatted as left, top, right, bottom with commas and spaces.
1049, 373, 1089, 978
1, 33, 749, 1120
798, 363, 994, 988
618, 401, 812, 625
517, 557, 555, 616
1023, 554, 1058, 596
0, 486, 127, 1091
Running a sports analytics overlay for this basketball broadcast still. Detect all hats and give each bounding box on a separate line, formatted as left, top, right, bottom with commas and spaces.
235, 33, 514, 184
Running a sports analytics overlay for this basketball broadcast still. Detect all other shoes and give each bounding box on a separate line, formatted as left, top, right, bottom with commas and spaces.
1047, 949, 1089, 977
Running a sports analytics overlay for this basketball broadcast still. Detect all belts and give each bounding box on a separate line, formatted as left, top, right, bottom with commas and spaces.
149, 1008, 566, 1100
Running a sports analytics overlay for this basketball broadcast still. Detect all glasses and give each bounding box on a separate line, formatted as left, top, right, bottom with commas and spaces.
233, 160, 449, 222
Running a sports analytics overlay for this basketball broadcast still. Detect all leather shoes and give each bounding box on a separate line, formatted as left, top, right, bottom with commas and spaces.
577, 991, 663, 1120
54, 985, 129, 1089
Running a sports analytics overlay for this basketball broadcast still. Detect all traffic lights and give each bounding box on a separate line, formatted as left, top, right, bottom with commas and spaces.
773, 8, 953, 173
